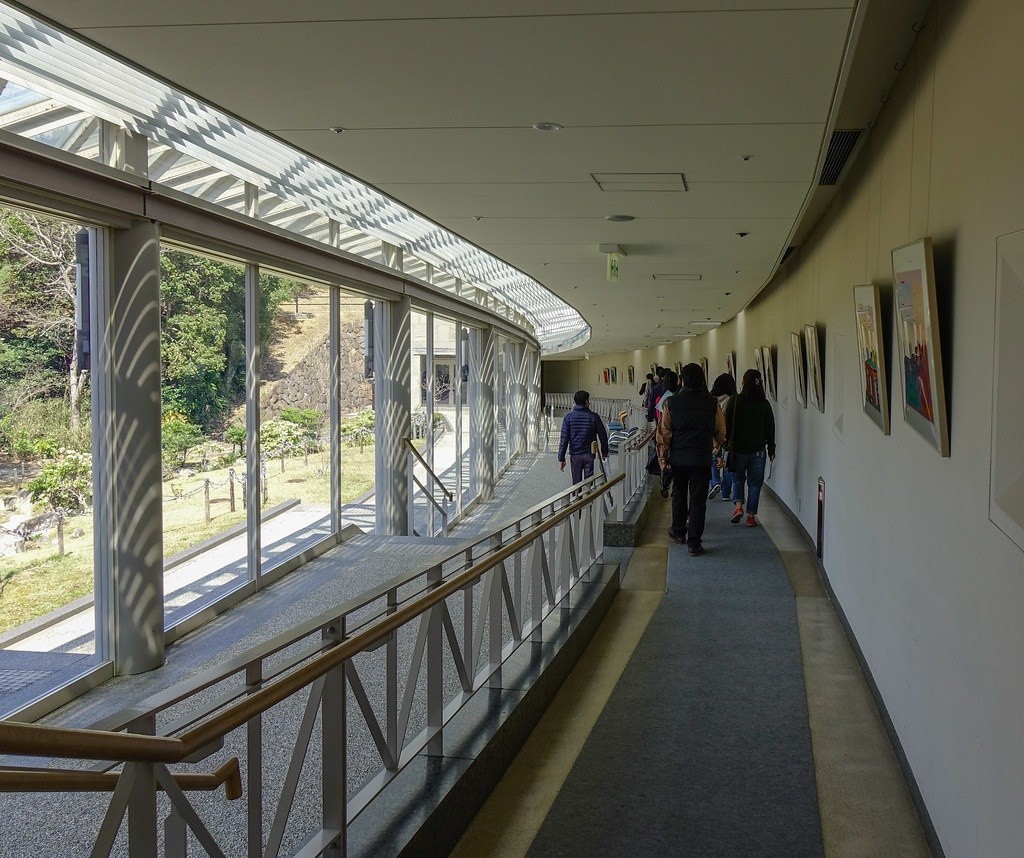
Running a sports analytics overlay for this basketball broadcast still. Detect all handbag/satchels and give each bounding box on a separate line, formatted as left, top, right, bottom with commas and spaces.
645, 448, 661, 476
724, 451, 735, 471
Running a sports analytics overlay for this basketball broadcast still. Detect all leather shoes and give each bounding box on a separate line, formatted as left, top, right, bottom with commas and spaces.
667, 528, 687, 545
688, 546, 708, 556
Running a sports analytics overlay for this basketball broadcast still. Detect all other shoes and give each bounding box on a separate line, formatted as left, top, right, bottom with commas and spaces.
723, 497, 730, 500
708, 484, 721, 500
729, 508, 744, 523
660, 490, 669, 498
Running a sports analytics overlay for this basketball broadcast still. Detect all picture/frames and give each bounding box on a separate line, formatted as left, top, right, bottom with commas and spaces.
603, 325, 824, 414
852, 282, 890, 437
888, 237, 950, 458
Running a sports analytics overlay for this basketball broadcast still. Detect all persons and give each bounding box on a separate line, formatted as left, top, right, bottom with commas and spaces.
558, 390, 609, 519
724, 369, 777, 526
656, 363, 726, 557
638, 366, 738, 502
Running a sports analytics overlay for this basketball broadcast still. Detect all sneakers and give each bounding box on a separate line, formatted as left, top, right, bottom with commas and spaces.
746, 515, 757, 526
